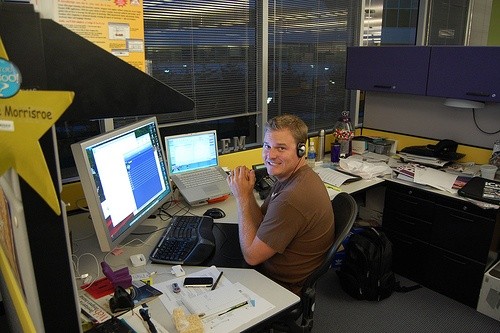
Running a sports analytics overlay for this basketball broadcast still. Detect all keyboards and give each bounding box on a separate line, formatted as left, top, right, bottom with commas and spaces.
148, 215, 216, 266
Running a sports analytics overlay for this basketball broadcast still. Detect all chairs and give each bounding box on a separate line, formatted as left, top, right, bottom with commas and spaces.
270, 192, 357, 333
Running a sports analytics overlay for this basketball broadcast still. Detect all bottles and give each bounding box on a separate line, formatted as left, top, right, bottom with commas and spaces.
331, 138, 341, 162
306, 142, 316, 168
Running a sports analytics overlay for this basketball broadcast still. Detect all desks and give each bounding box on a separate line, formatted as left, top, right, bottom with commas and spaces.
67, 151, 407, 333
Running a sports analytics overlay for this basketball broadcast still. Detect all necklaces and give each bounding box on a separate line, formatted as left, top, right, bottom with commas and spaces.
271, 158, 302, 199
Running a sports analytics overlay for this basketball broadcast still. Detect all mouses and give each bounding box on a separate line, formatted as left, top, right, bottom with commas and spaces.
202, 208, 226, 219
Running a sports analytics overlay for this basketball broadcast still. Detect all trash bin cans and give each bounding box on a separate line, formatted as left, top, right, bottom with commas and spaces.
476, 260, 500, 321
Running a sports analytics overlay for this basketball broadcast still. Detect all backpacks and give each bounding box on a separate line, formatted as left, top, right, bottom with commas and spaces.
333, 224, 422, 304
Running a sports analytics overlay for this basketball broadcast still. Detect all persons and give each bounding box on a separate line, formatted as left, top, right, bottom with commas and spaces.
226, 115, 335, 333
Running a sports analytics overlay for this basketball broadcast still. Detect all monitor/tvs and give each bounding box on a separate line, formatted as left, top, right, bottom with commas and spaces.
71, 115, 173, 253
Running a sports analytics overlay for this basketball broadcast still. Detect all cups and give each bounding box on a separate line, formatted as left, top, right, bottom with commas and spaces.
481, 164, 498, 180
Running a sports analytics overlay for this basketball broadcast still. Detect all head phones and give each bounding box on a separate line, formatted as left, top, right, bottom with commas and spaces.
296, 142, 307, 159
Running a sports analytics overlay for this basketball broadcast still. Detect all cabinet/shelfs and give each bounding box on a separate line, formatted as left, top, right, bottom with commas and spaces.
383, 172, 500, 310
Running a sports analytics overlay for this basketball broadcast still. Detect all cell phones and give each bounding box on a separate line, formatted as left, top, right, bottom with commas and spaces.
183, 277, 213, 288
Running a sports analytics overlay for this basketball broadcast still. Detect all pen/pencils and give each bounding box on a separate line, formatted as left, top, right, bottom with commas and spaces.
324, 182, 340, 191
217, 301, 248, 316
211, 271, 224, 291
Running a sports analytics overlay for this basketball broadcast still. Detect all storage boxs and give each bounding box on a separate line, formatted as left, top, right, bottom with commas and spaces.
345, 45, 500, 104
477, 260, 500, 322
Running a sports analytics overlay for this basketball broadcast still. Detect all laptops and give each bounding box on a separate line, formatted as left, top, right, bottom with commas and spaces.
164, 129, 231, 205
